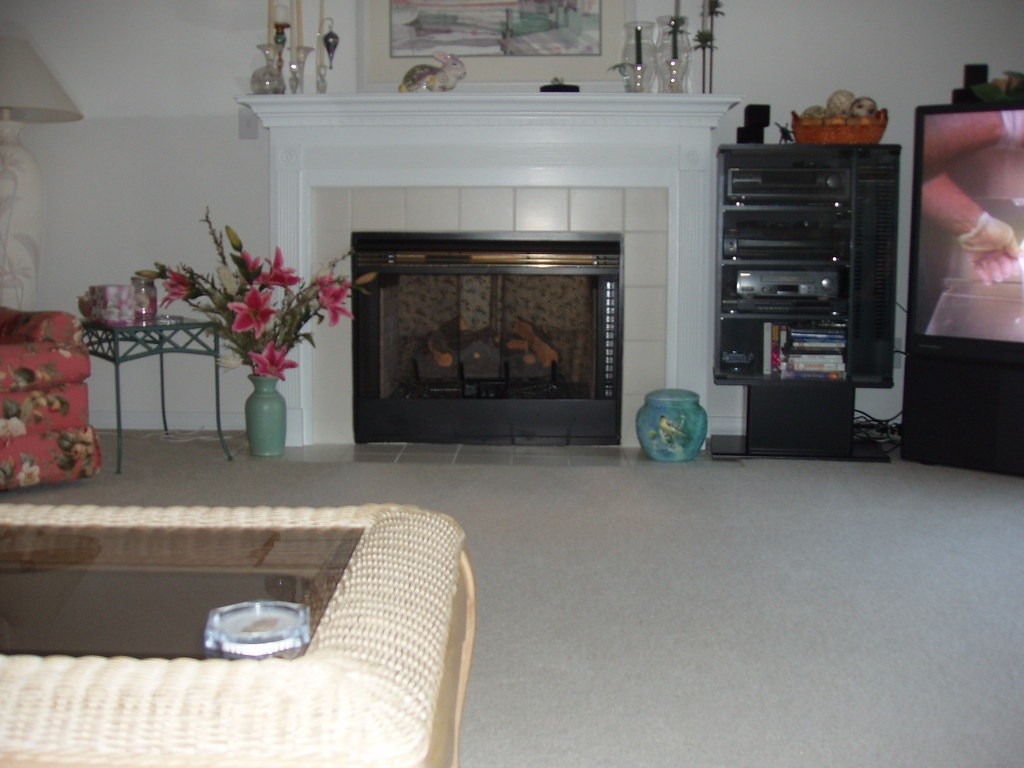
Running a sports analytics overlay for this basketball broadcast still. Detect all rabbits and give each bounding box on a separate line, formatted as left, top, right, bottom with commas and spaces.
401, 49, 467, 93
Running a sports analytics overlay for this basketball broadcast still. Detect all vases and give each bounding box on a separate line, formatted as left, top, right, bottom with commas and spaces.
652, 15, 691, 93
620, 21, 657, 94
245, 373, 287, 456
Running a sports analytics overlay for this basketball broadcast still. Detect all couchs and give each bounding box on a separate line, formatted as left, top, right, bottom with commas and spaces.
0, 305, 101, 490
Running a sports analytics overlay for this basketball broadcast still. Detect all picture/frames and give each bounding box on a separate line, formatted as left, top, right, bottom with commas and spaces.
355, 0, 637, 93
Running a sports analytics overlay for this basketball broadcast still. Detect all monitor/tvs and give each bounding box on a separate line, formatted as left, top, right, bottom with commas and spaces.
903, 101, 1024, 363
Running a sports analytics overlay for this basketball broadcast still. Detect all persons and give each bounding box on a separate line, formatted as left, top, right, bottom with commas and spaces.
922, 111, 1023, 306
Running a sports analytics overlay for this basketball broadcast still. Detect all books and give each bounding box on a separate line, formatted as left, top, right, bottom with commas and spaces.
759, 321, 848, 379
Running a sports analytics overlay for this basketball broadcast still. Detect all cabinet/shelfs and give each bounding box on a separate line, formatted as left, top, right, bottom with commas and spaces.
713, 144, 904, 453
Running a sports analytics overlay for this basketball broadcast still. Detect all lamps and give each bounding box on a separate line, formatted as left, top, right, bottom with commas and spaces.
0, 38, 85, 123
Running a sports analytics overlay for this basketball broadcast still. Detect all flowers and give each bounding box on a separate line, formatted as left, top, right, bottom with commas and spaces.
154, 205, 367, 381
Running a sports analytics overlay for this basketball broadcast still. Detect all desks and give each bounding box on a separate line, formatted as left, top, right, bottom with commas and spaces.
75, 312, 234, 474
0, 503, 477, 768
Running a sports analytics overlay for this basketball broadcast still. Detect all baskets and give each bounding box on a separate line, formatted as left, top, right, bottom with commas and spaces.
791, 109, 888, 144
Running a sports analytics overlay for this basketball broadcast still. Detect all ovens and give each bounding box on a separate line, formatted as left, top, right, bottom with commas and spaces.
350, 233, 624, 446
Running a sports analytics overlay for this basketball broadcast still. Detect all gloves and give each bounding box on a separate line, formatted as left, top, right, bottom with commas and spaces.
958, 212, 1020, 286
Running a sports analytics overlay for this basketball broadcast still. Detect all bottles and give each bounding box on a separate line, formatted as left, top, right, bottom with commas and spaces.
128, 277, 157, 319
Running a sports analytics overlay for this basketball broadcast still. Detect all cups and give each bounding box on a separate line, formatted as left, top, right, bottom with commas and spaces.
965, 65, 987, 111
